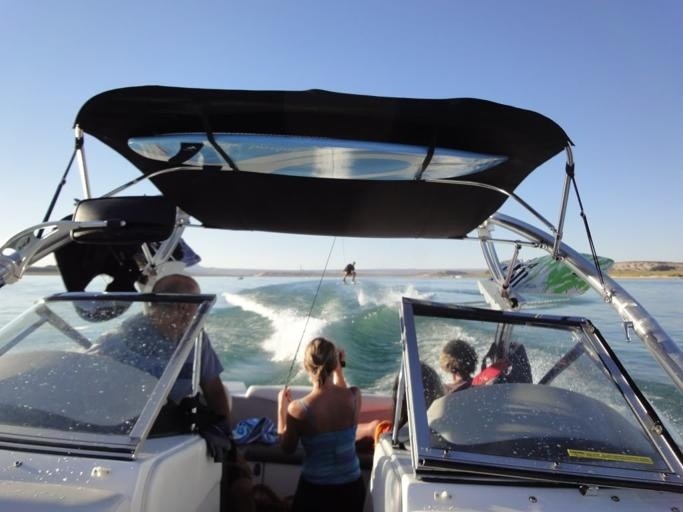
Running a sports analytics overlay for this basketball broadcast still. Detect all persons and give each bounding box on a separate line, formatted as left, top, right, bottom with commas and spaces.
439, 340, 478, 392
471, 338, 526, 387
87, 273, 252, 512
274, 337, 366, 512
353, 358, 443, 450
340, 261, 357, 285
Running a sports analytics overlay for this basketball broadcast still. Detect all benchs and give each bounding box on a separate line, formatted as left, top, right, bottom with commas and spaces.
228, 386, 394, 496
